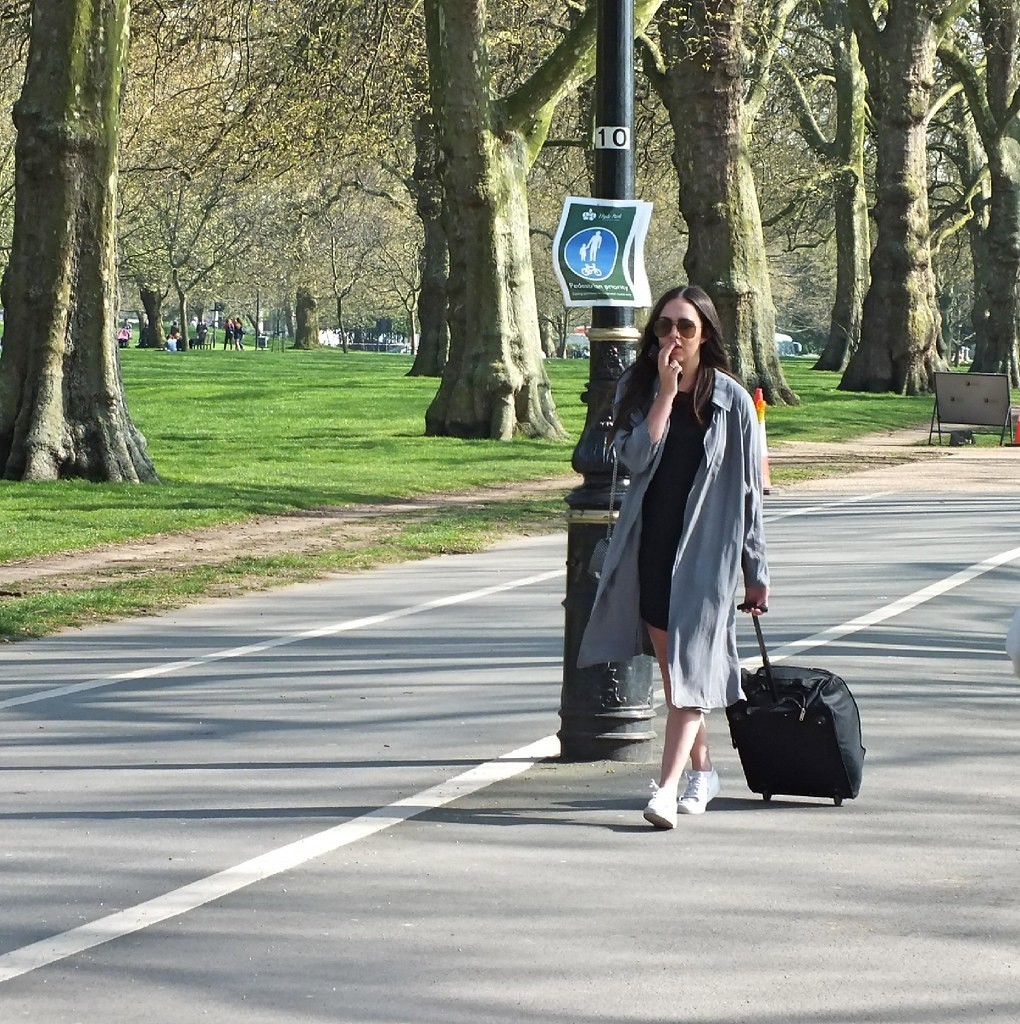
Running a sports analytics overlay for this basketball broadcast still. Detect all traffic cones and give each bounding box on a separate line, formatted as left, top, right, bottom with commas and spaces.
753, 387, 780, 495
1005, 413, 1020, 446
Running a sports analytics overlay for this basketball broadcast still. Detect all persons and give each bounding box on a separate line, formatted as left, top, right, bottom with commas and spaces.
118, 316, 244, 352
613, 286, 770, 828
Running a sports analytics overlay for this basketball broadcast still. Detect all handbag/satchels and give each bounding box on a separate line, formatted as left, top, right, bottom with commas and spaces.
587, 538, 609, 579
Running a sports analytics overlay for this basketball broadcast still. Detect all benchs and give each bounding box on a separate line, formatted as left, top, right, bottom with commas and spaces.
194, 333, 213, 349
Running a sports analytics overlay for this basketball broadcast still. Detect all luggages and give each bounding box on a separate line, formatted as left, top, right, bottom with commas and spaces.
725, 601, 867, 807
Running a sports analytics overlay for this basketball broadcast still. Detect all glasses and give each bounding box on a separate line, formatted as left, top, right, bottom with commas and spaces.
651, 318, 703, 340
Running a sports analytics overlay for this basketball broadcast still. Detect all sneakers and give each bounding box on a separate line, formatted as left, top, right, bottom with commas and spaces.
677, 770, 720, 815
643, 779, 677, 830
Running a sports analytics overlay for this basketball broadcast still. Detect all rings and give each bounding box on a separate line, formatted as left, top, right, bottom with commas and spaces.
668, 364, 675, 370
